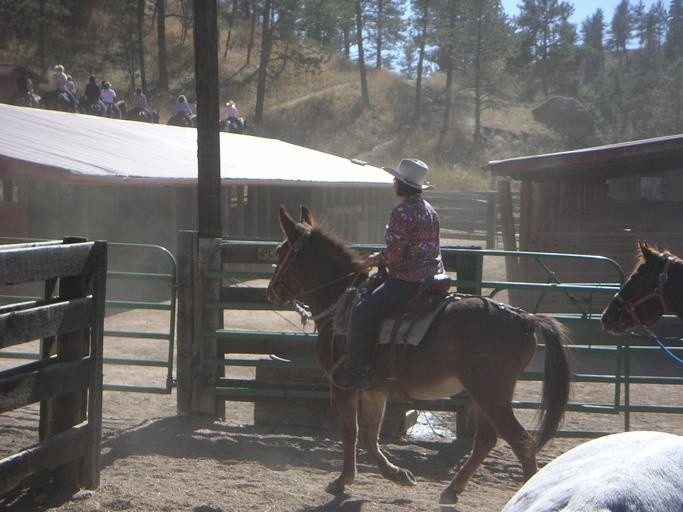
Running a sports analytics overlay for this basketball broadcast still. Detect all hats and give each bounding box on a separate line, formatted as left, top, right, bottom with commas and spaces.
382, 159, 434, 190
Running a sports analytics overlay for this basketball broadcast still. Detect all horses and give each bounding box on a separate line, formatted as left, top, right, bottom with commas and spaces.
21, 88, 249, 134
264, 204, 571, 506
600, 239, 683, 336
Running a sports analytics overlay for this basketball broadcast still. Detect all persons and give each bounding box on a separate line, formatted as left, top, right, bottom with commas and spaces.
334, 158, 445, 389
17, 64, 237, 129
